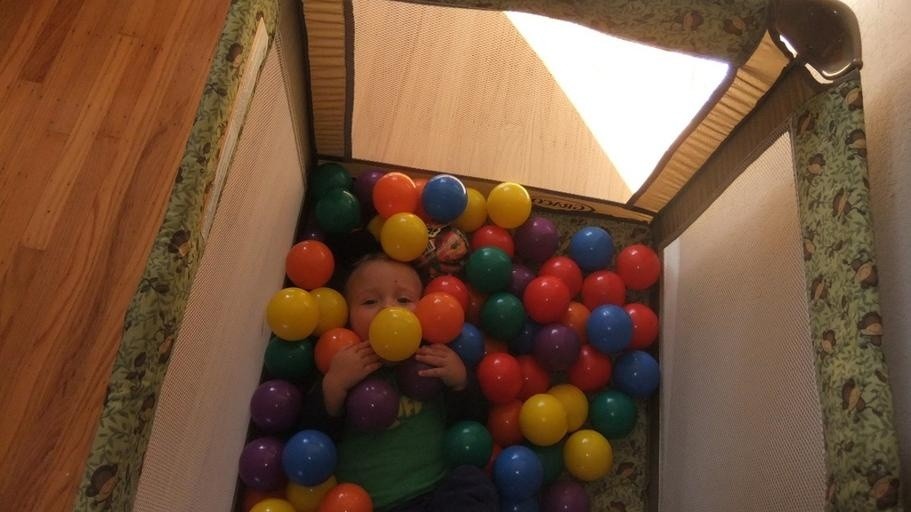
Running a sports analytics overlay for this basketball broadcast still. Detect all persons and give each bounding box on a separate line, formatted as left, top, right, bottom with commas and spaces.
318, 253, 499, 512
